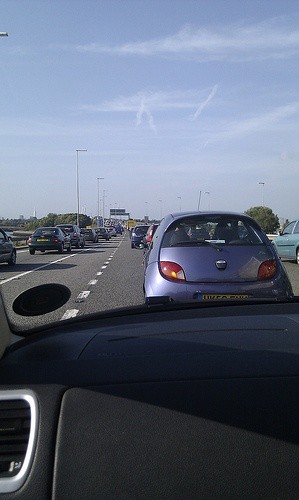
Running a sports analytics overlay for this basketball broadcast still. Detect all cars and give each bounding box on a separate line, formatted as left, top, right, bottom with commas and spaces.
130, 225, 150, 249
28, 226, 71, 255
271, 220, 299, 264
93, 227, 111, 241
106, 225, 124, 237
135, 210, 294, 305
80, 228, 99, 243
0, 228, 17, 266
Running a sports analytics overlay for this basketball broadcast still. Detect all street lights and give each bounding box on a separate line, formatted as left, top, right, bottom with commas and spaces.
96, 177, 104, 227
258, 182, 264, 207
75, 149, 88, 226
197, 190, 210, 210
175, 197, 181, 212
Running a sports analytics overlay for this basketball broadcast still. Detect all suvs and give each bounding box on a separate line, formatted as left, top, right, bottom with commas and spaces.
143, 224, 182, 255
54, 224, 86, 249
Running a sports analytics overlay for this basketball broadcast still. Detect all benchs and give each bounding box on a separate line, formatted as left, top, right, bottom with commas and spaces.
171, 227, 238, 243
